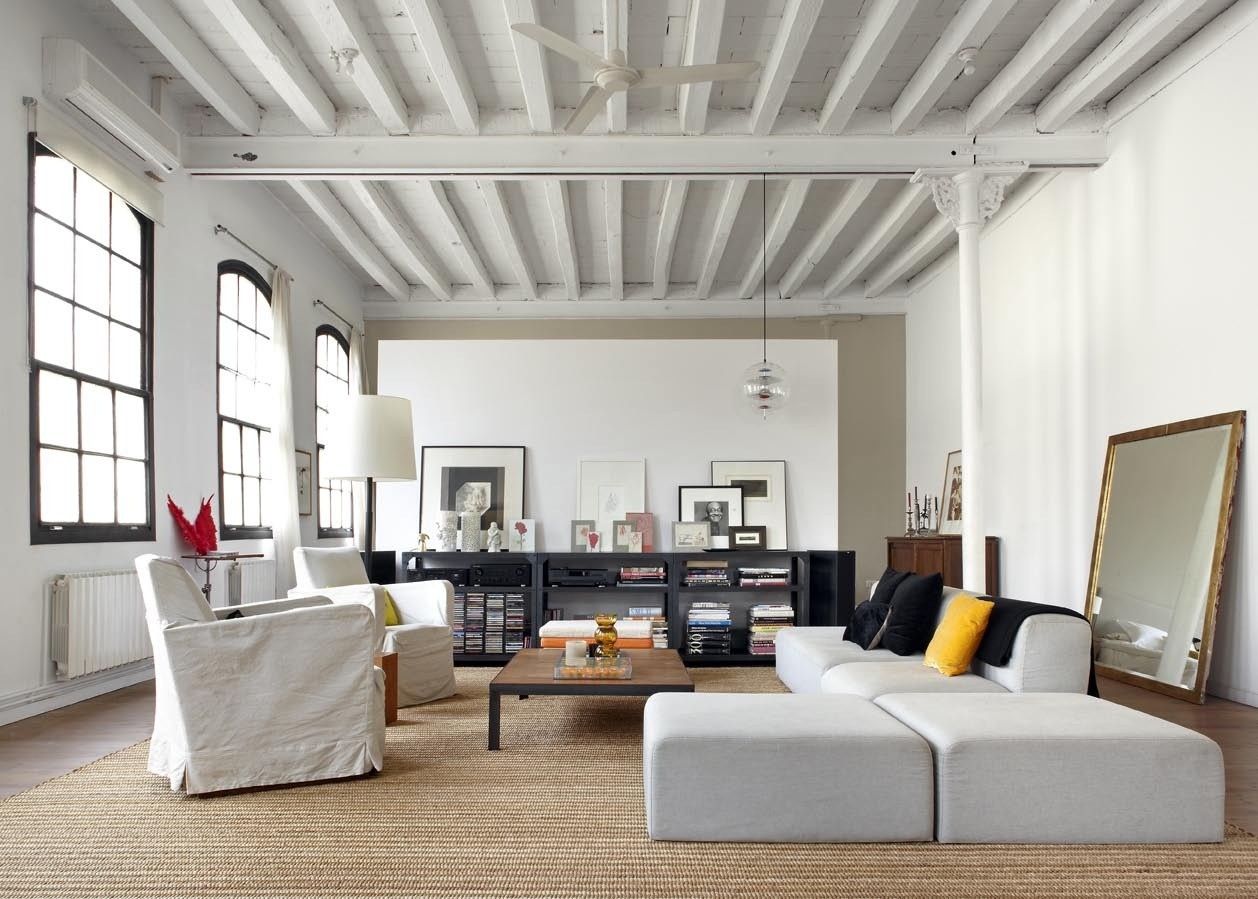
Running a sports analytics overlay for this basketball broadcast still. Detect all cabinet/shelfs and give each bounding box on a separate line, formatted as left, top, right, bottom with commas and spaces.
675, 548, 812, 662
884, 533, 962, 589
535, 550, 675, 651
401, 551, 536, 662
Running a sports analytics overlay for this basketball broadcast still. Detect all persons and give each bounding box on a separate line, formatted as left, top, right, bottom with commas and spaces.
486, 522, 504, 554
947, 466, 962, 521
700, 502, 725, 535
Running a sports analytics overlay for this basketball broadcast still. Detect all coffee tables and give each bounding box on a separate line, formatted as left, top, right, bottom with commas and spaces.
486, 650, 695, 752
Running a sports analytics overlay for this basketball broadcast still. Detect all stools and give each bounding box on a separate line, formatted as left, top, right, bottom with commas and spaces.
539, 620, 654, 650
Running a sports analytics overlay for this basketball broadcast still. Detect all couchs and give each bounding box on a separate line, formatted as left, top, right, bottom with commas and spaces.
289, 545, 459, 705
134, 554, 390, 793
644, 580, 1227, 847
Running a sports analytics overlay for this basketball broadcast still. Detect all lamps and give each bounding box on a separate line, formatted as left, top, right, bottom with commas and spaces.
740, 174, 792, 420
326, 392, 418, 582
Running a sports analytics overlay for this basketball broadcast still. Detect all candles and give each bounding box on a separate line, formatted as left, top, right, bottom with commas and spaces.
923, 494, 927, 508
935, 497, 938, 510
914, 486, 918, 499
907, 492, 911, 506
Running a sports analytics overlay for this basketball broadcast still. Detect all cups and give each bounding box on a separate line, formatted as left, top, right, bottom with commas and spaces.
565, 639, 590, 666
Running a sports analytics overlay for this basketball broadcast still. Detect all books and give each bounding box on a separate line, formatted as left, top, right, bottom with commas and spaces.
684, 601, 795, 655
453, 592, 532, 655
615, 566, 667, 585
542, 608, 563, 621
621, 606, 669, 650
680, 559, 791, 587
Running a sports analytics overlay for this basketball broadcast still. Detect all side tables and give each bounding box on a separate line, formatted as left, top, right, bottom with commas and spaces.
379, 652, 398, 724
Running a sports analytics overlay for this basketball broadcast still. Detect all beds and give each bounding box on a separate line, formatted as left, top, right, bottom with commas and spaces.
1100, 637, 1198, 692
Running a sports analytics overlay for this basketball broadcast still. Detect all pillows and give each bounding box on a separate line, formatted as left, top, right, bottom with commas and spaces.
924, 591, 995, 675
843, 600, 894, 646
1116, 619, 1195, 654
870, 566, 910, 600
885, 571, 944, 655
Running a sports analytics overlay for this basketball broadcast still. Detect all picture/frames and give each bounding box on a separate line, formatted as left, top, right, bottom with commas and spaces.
294, 449, 313, 517
413, 442, 791, 553
936, 449, 963, 536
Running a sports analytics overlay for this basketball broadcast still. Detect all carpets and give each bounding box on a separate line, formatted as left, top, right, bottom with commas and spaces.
1, 667, 1258, 899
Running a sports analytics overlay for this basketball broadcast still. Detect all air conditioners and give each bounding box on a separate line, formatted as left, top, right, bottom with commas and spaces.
40, 34, 181, 184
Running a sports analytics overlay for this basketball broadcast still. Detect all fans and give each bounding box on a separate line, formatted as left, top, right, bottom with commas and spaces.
511, 1, 754, 135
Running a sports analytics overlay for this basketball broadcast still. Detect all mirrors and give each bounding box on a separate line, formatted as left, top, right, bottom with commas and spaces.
1082, 409, 1246, 707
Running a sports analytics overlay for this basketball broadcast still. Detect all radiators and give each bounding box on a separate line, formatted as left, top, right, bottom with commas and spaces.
228, 559, 278, 605
50, 570, 154, 682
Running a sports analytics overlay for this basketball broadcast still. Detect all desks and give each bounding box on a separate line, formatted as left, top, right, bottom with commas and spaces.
181, 550, 266, 603
